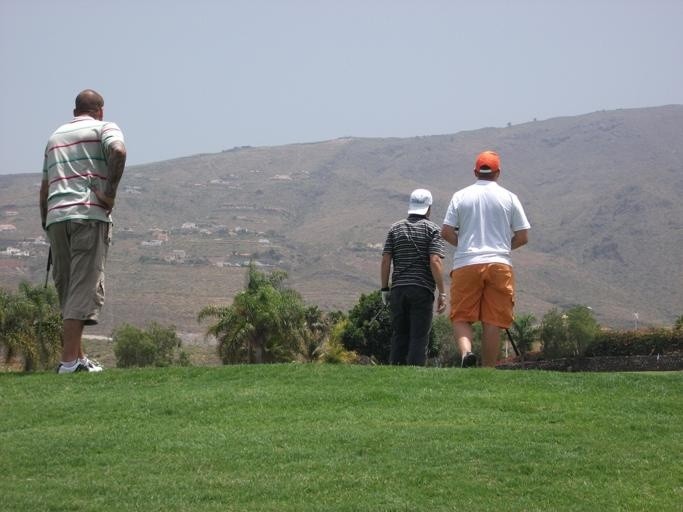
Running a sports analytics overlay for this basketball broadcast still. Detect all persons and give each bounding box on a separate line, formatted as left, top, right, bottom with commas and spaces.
379, 187, 446, 367
438, 149, 533, 369
36, 88, 127, 375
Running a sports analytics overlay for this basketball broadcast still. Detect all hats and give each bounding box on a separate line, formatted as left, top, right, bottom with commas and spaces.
472, 150, 501, 175
406, 188, 433, 218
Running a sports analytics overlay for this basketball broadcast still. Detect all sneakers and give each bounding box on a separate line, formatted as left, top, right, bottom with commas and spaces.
460, 351, 479, 367
56, 357, 103, 375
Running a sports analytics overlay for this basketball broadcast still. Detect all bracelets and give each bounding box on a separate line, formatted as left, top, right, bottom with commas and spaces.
381, 286, 389, 291
438, 292, 446, 297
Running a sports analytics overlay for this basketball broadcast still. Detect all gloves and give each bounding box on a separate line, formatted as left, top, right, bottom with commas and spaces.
380, 286, 390, 306
